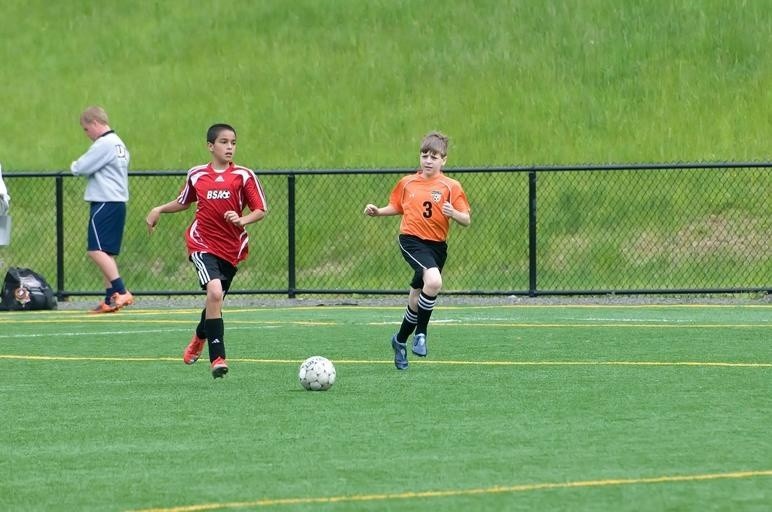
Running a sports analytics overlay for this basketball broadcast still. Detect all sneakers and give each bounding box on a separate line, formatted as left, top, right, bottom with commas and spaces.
392, 333, 409, 370
210, 356, 228, 379
86, 290, 134, 314
183, 332, 206, 365
412, 328, 427, 357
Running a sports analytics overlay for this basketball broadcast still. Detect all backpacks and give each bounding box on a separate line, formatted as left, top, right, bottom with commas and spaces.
0, 266, 57, 311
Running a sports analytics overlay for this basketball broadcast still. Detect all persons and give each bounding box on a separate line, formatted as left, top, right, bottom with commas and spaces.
361, 131, 472, 371
143, 122, 268, 378
68, 105, 135, 315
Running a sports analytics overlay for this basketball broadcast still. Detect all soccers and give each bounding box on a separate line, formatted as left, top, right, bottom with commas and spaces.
299, 355, 336, 391
